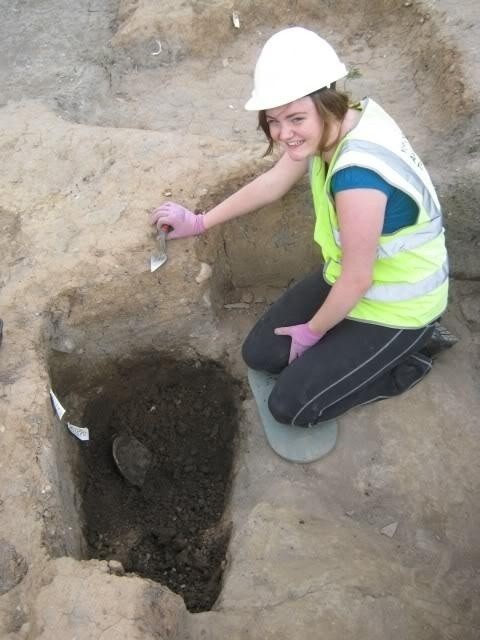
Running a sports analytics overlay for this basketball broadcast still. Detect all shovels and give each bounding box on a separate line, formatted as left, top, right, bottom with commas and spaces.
151, 224, 169, 273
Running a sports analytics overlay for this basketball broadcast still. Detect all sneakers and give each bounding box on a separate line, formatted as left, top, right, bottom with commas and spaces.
419, 321, 461, 359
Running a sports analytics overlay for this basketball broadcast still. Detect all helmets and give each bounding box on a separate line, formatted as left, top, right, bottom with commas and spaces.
244, 26, 348, 111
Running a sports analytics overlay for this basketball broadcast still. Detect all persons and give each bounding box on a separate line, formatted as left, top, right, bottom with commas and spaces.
145, 25, 463, 430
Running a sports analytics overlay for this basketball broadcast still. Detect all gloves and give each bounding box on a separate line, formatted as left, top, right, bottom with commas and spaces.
149, 202, 208, 241
273, 321, 326, 367
